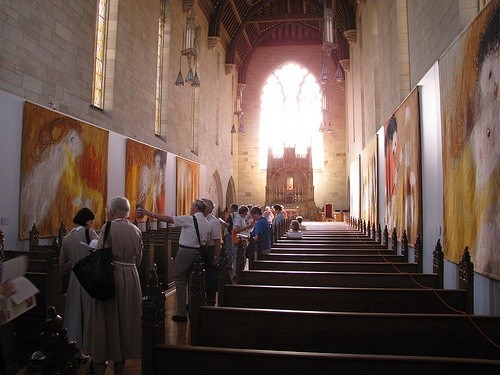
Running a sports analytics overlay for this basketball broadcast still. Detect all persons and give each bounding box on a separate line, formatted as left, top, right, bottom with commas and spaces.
87, 195, 144, 375
58, 207, 108, 375
136, 199, 211, 322
218, 202, 288, 277
185, 198, 222, 310
296, 216, 306, 230
0, 277, 16, 307
287, 220, 303, 239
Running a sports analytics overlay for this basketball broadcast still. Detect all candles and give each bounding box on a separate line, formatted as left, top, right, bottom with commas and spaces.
275, 185, 277, 198
282, 186, 285, 199
298, 186, 300, 198
279, 186, 280, 198
302, 186, 304, 195
294, 186, 296, 198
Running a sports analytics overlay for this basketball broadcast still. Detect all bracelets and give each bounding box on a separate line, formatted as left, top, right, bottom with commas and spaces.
151, 213, 155, 217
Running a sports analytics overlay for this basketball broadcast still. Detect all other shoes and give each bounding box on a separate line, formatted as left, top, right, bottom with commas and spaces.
173, 315, 188, 324
81, 356, 89, 362
190, 318, 199, 324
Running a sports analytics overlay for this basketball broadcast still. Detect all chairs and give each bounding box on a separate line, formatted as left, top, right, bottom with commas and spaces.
324, 203, 335, 222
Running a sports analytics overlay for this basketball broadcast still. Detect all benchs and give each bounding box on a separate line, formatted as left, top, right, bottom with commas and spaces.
0, 209, 500, 375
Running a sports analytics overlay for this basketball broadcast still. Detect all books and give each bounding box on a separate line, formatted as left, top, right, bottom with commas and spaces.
0, 256, 40, 303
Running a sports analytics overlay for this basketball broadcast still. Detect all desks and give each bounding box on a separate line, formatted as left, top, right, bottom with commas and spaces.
336, 213, 344, 222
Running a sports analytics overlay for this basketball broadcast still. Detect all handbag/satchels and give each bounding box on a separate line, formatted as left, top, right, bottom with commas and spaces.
218, 244, 228, 272
72, 221, 117, 300
228, 221, 233, 232
245, 238, 255, 260
199, 245, 210, 260
232, 236, 241, 245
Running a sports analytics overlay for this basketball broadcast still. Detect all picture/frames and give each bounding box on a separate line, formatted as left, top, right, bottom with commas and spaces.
124, 137, 167, 225
349, 133, 378, 231
437, 0, 500, 283
175, 156, 201, 217
382, 84, 423, 250
18, 101, 110, 242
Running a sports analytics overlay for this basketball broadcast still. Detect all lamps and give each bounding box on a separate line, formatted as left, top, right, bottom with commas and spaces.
176, 0, 344, 132
